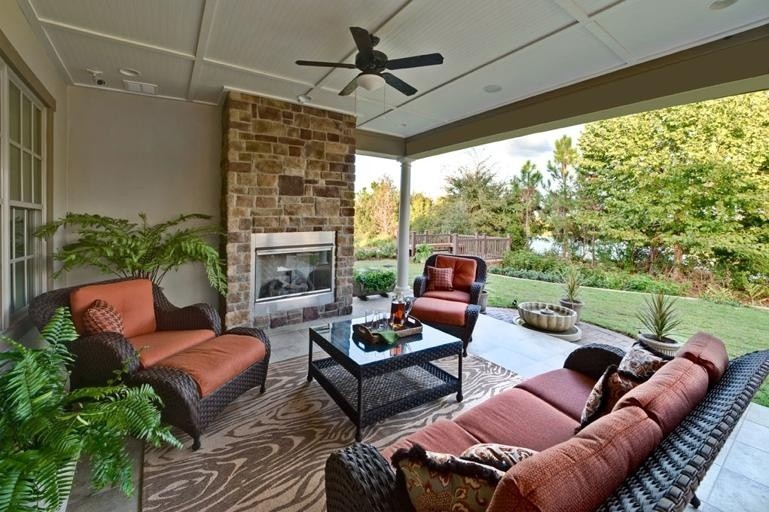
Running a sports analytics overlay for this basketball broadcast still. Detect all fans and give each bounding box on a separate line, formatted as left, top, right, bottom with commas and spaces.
293, 24, 445, 119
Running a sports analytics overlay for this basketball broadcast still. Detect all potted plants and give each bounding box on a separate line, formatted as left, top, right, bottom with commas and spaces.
558, 271, 586, 326
634, 287, 684, 357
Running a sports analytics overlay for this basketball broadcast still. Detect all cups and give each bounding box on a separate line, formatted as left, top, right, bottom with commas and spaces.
376, 310, 389, 332
365, 312, 374, 329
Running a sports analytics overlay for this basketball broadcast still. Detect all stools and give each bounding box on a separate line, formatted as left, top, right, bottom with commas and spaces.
129, 326, 271, 450
391, 296, 482, 358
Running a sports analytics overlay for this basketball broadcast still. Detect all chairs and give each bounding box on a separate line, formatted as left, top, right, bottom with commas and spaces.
412, 253, 487, 343
29, 275, 221, 403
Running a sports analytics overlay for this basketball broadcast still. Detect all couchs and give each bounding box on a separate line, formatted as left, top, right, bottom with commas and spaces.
324, 331, 768, 512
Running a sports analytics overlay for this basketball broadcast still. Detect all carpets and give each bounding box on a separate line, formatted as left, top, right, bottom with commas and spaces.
142, 351, 527, 512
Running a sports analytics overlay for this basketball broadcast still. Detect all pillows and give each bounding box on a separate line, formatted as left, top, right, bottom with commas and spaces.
389, 444, 539, 512
574, 343, 671, 432
426, 264, 456, 292
81, 299, 126, 340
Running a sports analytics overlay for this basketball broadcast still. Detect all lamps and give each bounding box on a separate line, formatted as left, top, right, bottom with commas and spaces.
356, 71, 386, 93
